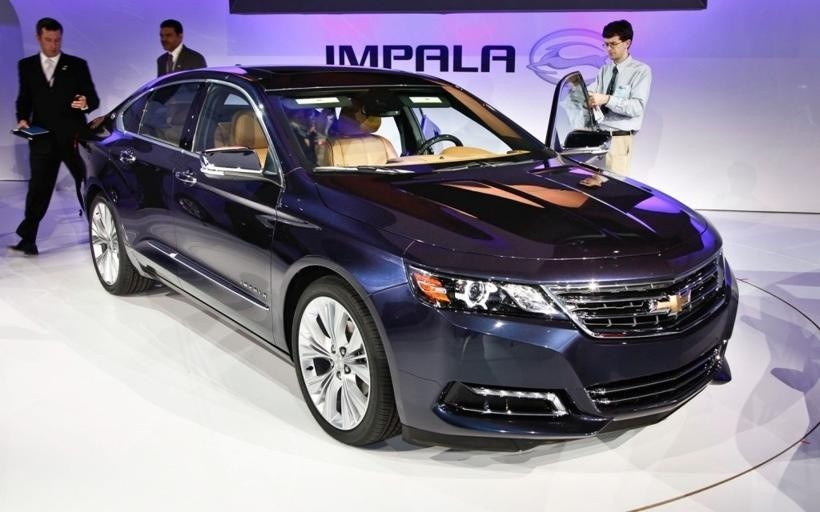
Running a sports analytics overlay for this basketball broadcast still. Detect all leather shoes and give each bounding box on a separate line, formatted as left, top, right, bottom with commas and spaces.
5, 238, 40, 258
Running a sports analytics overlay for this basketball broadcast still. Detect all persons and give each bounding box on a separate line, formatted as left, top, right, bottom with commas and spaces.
4, 16, 100, 256
585, 18, 654, 175
156, 18, 208, 77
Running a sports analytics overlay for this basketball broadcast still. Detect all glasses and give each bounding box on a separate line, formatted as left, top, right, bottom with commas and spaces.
601, 40, 625, 48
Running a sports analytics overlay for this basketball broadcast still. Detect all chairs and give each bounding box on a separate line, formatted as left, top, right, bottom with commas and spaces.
228, 109, 268, 168
325, 100, 397, 167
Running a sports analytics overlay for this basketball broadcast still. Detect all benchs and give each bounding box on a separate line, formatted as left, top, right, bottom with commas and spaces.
162, 120, 329, 169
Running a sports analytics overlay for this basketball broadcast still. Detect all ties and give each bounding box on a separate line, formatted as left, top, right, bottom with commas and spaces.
45, 60, 55, 84
167, 54, 176, 73
599, 66, 619, 117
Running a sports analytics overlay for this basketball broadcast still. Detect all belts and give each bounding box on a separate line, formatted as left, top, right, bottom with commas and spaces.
598, 128, 640, 138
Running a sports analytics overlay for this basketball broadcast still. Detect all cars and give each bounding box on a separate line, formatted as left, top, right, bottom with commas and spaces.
56, 60, 742, 453
